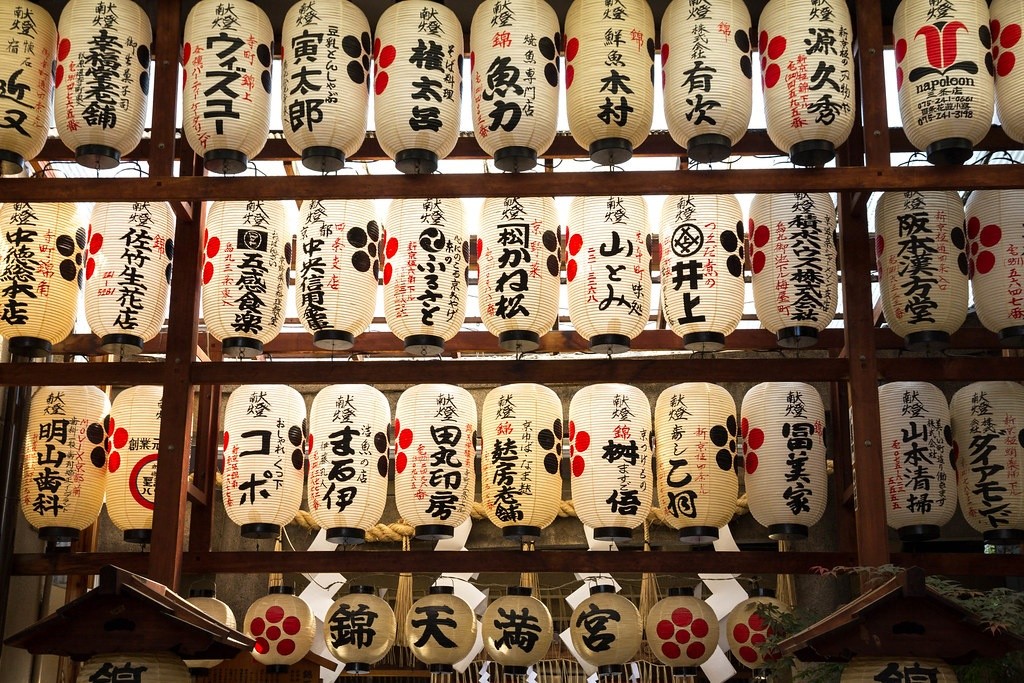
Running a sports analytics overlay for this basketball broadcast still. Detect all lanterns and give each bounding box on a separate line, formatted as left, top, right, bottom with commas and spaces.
0, 0, 1024, 683
